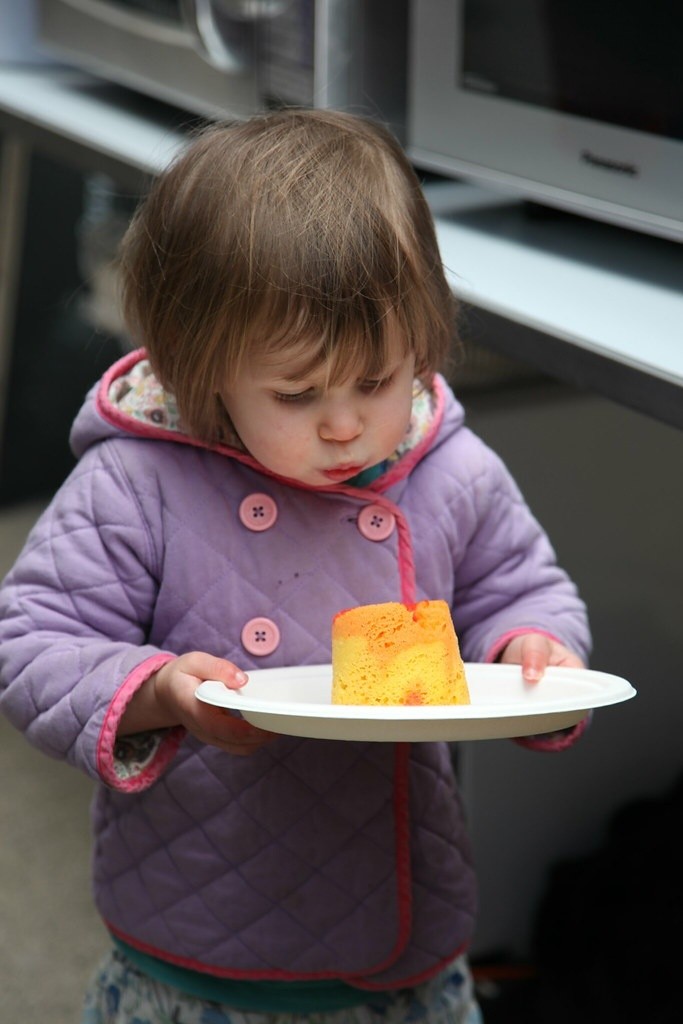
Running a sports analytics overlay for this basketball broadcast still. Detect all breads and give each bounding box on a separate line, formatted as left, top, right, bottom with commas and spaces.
328, 600, 470, 708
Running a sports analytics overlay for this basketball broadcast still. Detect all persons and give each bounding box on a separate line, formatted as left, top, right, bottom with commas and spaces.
0, 110, 594, 1024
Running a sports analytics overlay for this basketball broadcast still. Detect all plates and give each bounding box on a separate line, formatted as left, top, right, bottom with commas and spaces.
194, 663, 637, 742
